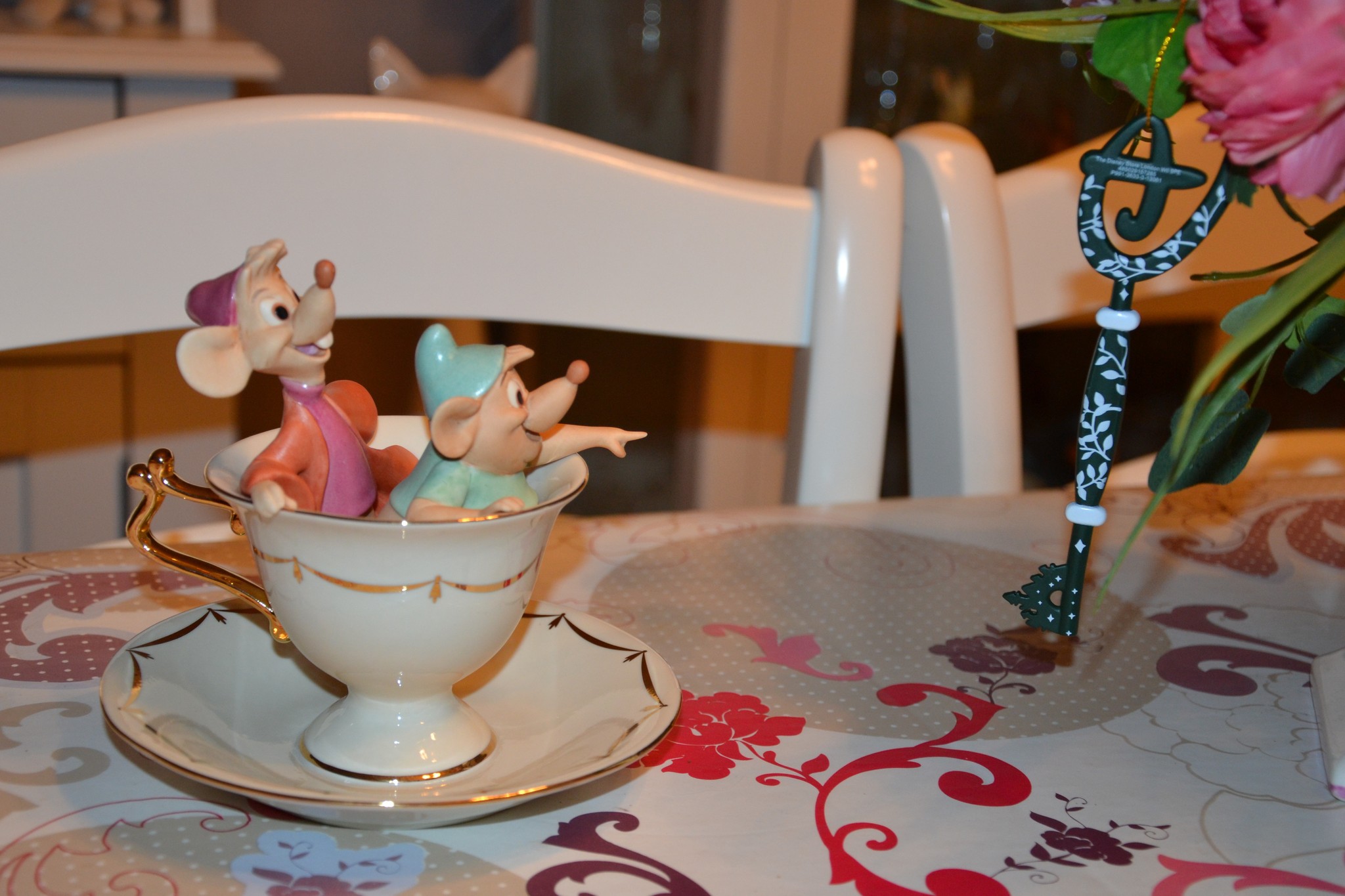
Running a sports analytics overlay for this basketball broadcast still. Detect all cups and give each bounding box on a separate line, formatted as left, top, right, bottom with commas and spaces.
121, 416, 596, 782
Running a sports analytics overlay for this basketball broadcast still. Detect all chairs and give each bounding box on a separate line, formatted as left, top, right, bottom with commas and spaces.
892, 102, 1345, 496
0, 94, 905, 535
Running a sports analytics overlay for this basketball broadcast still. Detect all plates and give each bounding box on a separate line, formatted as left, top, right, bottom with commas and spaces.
97, 603, 680, 826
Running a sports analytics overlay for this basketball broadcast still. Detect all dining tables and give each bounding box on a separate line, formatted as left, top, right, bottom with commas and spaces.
1, 467, 1345, 896
